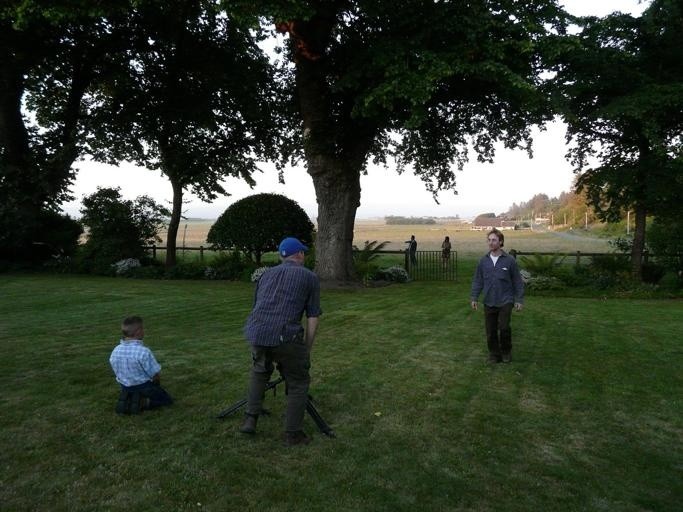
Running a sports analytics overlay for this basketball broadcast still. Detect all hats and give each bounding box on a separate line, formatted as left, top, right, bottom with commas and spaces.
279, 237, 309, 258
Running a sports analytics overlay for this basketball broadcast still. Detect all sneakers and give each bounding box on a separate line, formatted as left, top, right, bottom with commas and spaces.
282, 432, 311, 447
115, 390, 145, 416
489, 351, 512, 363
239, 416, 258, 434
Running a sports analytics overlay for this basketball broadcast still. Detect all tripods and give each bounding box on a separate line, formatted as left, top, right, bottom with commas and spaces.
218, 375, 336, 437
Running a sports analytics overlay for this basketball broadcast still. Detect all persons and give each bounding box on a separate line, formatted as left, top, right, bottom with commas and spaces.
470, 226, 525, 367
239, 236, 325, 443
440, 236, 451, 272
106, 313, 176, 417
403, 235, 418, 270
